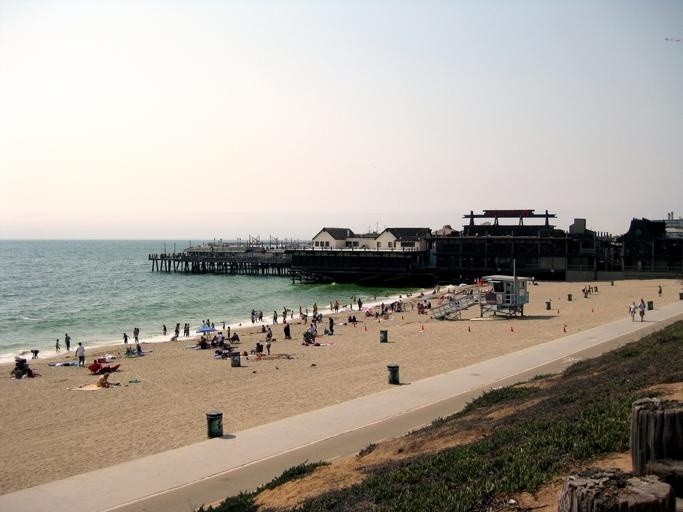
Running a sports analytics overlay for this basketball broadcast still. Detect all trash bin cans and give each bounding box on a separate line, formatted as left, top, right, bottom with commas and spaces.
594, 287, 598, 292
611, 281, 614, 286
568, 294, 572, 301
386, 364, 399, 384
680, 293, 683, 300
545, 302, 551, 310
206, 412, 223, 439
647, 301, 653, 310
380, 330, 387, 343
230, 352, 240, 367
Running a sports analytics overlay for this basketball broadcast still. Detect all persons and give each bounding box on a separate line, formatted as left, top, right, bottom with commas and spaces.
638, 298, 645, 322
65, 334, 71, 352
97, 373, 108, 387
89, 360, 101, 374
124, 285, 473, 360
532, 276, 535, 282
657, 285, 662, 298
31, 350, 39, 359
10, 358, 40, 378
56, 339, 60, 351
629, 301, 636, 321
75, 342, 86, 367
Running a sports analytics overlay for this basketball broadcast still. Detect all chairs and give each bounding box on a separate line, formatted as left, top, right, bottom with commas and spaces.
200, 335, 238, 349
